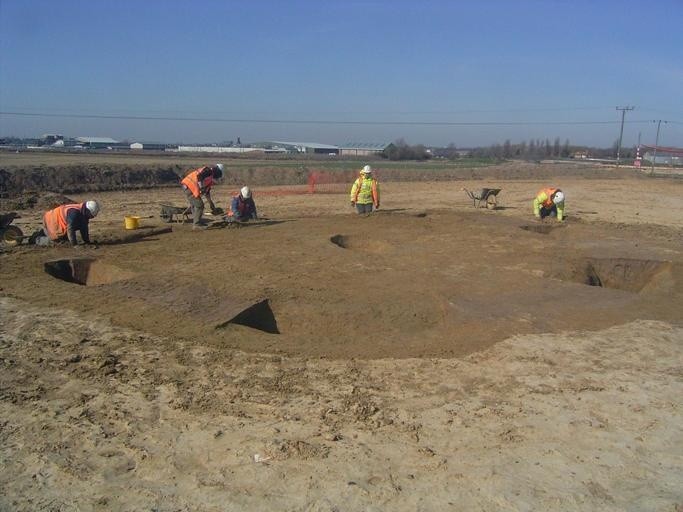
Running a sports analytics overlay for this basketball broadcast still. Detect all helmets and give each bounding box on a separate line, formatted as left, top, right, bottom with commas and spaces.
85, 199, 98, 218
216, 161, 224, 177
240, 186, 251, 199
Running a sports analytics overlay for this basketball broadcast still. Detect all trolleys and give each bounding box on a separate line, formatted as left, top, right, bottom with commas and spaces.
461, 187, 501, 210
0, 212, 22, 246
159, 203, 191, 223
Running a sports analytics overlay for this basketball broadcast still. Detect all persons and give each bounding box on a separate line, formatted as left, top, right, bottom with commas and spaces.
43, 201, 100, 246
350, 164, 380, 215
181, 163, 225, 224
228, 185, 258, 224
533, 186, 565, 221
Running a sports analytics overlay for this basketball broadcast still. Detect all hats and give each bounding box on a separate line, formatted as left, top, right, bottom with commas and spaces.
553, 191, 564, 205
361, 165, 372, 173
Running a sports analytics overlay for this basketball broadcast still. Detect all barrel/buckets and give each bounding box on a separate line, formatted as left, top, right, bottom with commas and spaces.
124, 215, 139, 229
